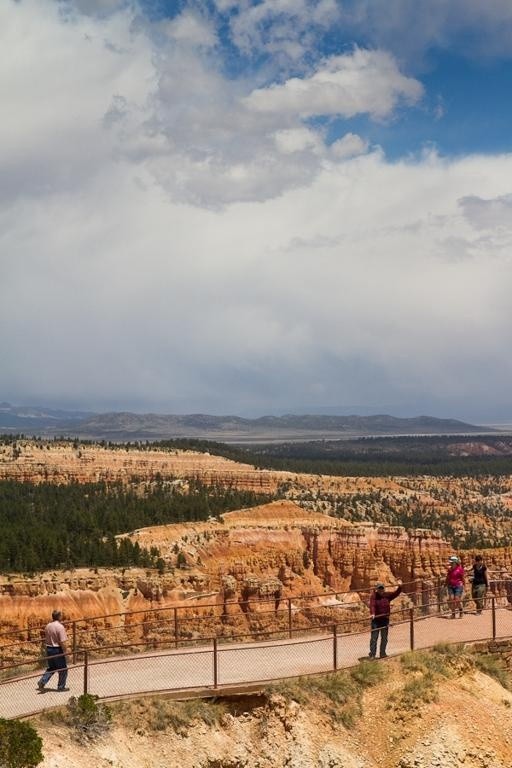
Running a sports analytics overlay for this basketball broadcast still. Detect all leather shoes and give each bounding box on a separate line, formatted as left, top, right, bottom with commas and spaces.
38, 680, 45, 693
57, 687, 69, 692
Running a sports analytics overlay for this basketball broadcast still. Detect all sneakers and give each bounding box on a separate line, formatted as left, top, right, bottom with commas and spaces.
447, 614, 455, 618
459, 608, 464, 617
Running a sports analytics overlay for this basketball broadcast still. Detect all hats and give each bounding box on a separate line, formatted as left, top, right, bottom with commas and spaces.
377, 582, 385, 589
448, 557, 459, 563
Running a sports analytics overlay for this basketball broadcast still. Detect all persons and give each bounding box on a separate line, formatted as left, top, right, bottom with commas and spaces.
369, 579, 403, 659
38, 608, 71, 692
470, 555, 488, 617
443, 556, 463, 620
